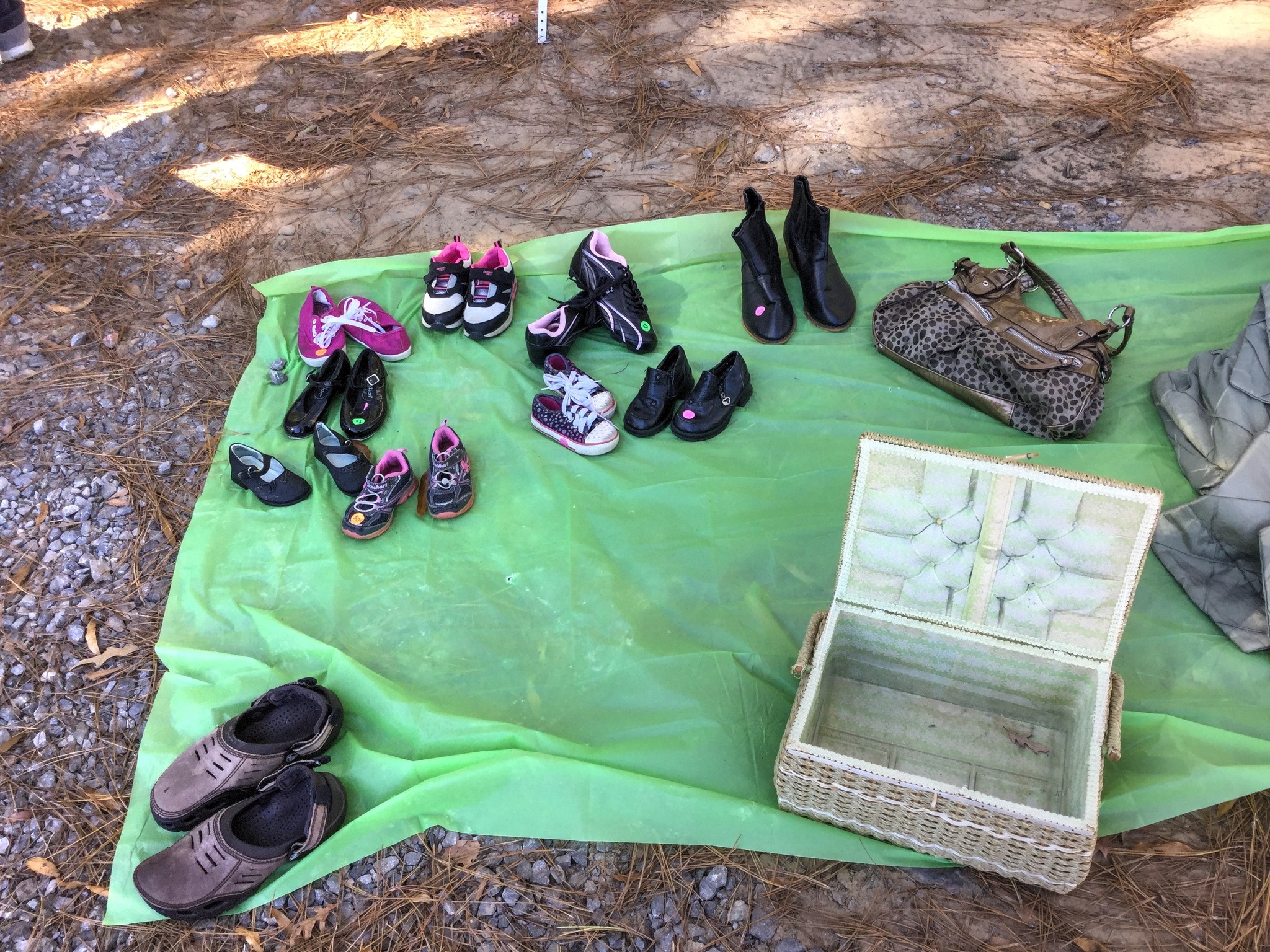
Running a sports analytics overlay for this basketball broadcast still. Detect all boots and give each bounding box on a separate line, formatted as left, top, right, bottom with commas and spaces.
783, 175, 857, 332
731, 187, 797, 345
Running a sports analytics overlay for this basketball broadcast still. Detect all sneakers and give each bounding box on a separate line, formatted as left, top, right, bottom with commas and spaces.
543, 353, 616, 421
525, 289, 602, 370
0, 23, 36, 69
342, 448, 420, 540
134, 764, 350, 922
421, 241, 473, 334
530, 393, 620, 455
426, 418, 475, 519
297, 285, 348, 368
149, 677, 345, 834
463, 247, 517, 340
336, 296, 412, 362
566, 229, 658, 354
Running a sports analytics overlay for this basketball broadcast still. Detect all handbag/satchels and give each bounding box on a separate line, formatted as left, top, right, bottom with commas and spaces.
865, 240, 1135, 441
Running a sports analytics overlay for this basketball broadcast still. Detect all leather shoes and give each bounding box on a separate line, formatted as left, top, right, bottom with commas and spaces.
313, 421, 375, 497
339, 349, 388, 440
671, 351, 754, 442
229, 444, 313, 507
283, 347, 351, 439
623, 345, 695, 438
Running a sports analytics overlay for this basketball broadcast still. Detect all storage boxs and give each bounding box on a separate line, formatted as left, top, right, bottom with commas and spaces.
778, 429, 1166, 898
